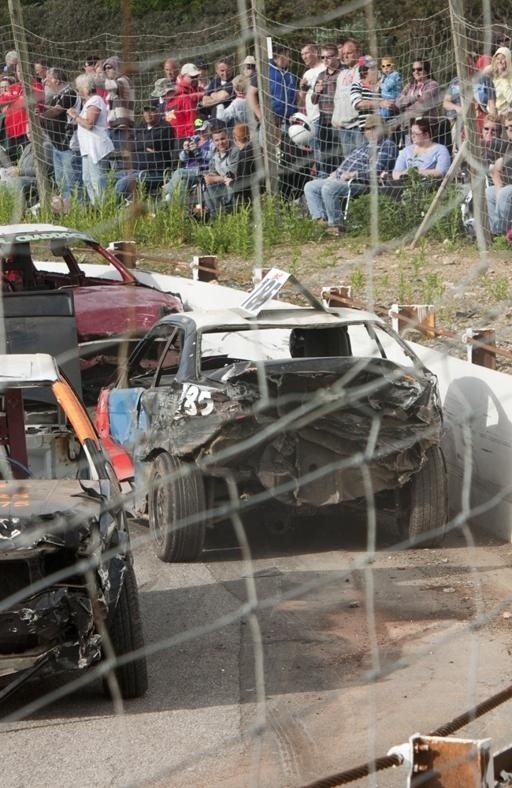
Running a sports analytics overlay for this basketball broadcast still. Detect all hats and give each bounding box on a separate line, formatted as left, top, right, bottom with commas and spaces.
150, 77, 177, 98
180, 63, 202, 77
365, 115, 386, 130
192, 113, 213, 132
142, 98, 160, 110
238, 55, 257, 67
103, 56, 123, 74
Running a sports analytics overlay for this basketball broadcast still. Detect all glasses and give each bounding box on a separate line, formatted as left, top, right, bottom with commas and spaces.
503, 124, 512, 130
410, 67, 423, 72
483, 126, 497, 131
380, 63, 396, 68
320, 53, 336, 59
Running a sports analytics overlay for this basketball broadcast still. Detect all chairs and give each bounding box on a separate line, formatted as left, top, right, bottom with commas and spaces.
342, 175, 465, 220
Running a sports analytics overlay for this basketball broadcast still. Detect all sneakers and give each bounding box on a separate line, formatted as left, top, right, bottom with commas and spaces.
315, 218, 328, 229
325, 226, 345, 239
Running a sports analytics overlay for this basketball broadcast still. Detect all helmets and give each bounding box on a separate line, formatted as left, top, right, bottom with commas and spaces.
287, 112, 316, 147
